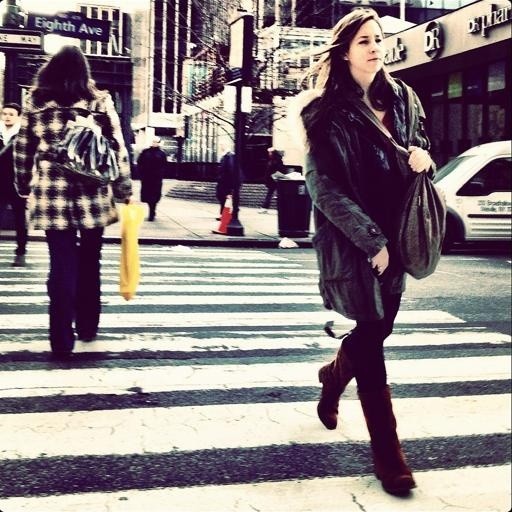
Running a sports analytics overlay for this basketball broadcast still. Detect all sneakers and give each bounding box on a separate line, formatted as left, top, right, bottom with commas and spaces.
49, 325, 97, 360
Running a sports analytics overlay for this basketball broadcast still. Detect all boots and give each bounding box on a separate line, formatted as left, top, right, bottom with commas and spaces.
317, 336, 358, 430
357, 384, 417, 495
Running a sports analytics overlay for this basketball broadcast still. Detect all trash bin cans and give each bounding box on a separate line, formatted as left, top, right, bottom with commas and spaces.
276, 179, 311, 239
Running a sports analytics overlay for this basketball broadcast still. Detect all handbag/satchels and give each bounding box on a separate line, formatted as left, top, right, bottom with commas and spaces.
53, 99, 120, 187
398, 146, 446, 279
116, 203, 144, 301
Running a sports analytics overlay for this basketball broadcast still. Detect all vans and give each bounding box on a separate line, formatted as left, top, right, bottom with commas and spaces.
431, 140, 512, 254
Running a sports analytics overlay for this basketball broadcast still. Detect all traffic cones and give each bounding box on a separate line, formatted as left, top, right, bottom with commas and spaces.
212, 194, 233, 235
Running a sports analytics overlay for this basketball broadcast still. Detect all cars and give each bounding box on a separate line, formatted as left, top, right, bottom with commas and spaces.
159, 139, 178, 155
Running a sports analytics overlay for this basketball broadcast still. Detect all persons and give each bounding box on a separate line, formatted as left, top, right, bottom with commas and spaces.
1, 103, 28, 266
15, 45, 133, 352
299, 9, 436, 493
216, 140, 239, 220
137, 137, 167, 221
258, 147, 285, 213
